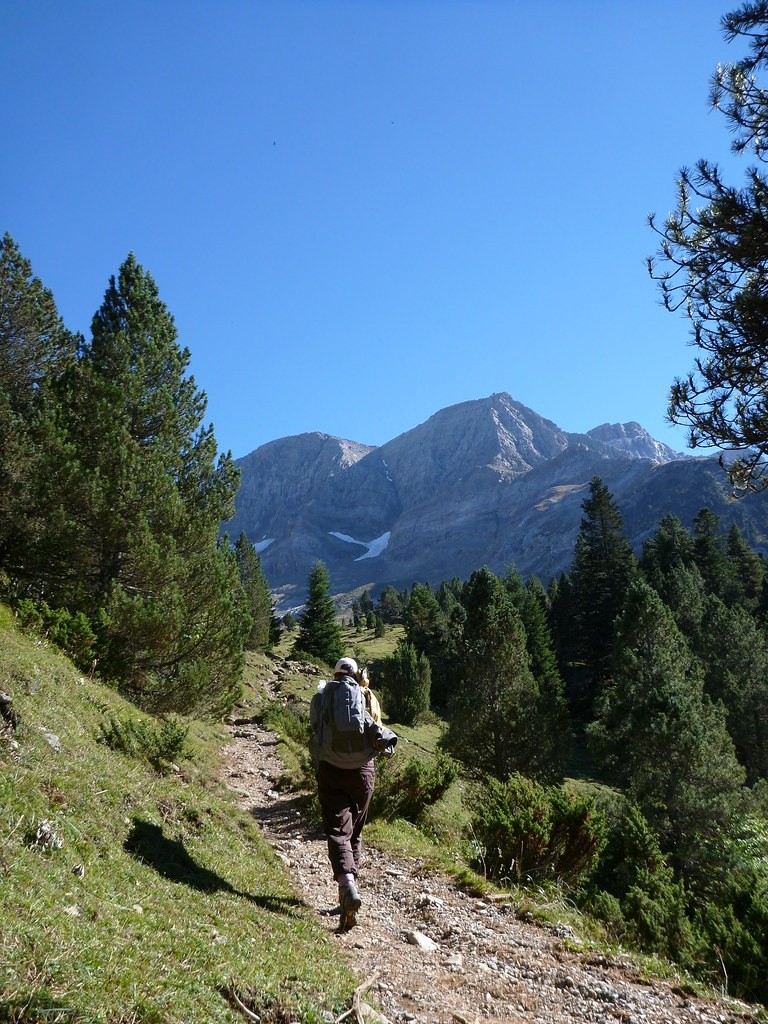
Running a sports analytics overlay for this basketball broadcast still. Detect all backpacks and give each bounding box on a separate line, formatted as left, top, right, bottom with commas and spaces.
317, 678, 374, 756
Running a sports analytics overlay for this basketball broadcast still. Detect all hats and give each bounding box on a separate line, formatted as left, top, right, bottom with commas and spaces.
333, 658, 360, 677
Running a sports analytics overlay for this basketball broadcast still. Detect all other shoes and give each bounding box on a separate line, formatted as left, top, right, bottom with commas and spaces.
338, 883, 360, 935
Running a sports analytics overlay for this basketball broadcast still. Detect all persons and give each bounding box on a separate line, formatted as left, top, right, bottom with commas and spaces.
305, 657, 382, 933
